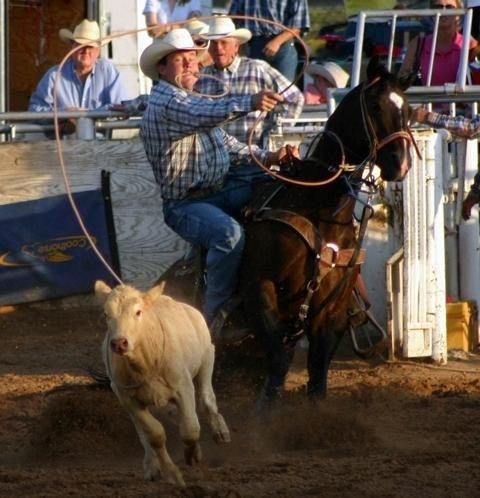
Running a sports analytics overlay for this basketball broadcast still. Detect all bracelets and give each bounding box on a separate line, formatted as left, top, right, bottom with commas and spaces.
424, 112, 430, 122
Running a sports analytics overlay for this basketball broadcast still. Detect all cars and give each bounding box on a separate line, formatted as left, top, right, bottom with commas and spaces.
320, 17, 424, 57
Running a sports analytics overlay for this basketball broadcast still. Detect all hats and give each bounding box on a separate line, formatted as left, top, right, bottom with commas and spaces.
306, 58, 351, 90
183, 17, 209, 35
59, 20, 111, 50
137, 28, 207, 81
199, 13, 253, 46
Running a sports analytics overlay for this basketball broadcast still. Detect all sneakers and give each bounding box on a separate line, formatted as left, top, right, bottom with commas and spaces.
211, 322, 247, 345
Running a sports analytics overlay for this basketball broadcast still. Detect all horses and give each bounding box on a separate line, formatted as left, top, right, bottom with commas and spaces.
84, 52, 421, 400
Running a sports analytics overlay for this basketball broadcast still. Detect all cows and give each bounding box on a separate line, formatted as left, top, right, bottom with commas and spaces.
93, 278, 232, 491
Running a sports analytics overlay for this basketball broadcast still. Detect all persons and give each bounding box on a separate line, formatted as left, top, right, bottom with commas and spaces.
27, 19, 125, 141
108, 13, 306, 154
137, 26, 298, 348
226, 0, 311, 87
411, 105, 480, 220
395, 0, 480, 115
140, 0, 216, 40
